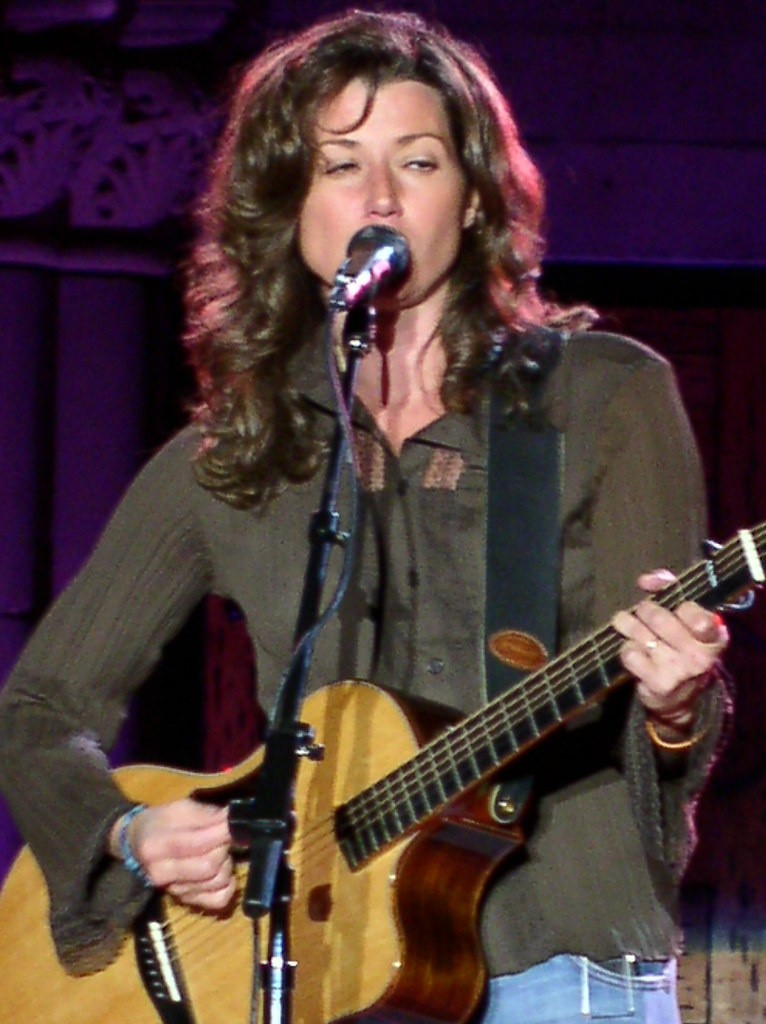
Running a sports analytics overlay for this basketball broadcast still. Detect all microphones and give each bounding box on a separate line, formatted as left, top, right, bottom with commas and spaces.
328, 224, 412, 313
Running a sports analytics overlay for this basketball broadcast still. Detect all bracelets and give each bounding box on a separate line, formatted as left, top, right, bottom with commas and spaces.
645, 686, 717, 752
119, 804, 152, 889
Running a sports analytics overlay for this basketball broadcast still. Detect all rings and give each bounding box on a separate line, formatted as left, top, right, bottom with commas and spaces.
645, 639, 660, 649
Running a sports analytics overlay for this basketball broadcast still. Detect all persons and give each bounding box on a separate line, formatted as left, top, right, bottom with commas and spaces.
0, 7, 733, 1024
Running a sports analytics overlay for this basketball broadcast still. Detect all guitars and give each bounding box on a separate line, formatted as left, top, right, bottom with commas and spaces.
0, 517, 765, 1024
